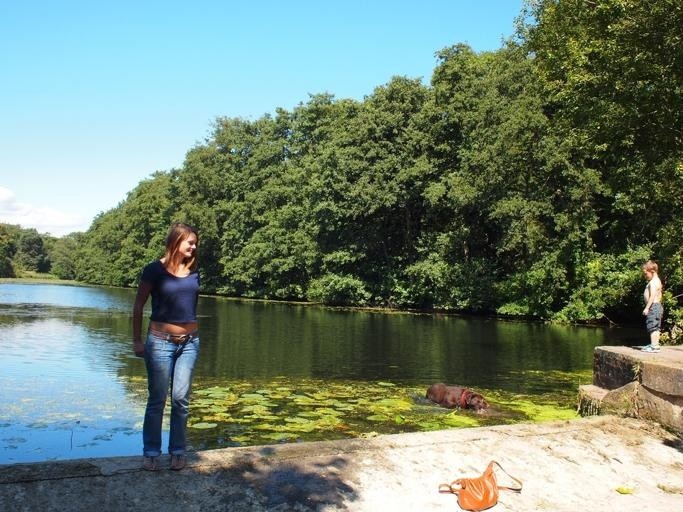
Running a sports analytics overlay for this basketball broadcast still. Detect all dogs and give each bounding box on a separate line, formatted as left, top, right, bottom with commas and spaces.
424, 383, 490, 411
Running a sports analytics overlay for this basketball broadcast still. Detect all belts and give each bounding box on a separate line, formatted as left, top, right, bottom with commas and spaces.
148, 329, 198, 344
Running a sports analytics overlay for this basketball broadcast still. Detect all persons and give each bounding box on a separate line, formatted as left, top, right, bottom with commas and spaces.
131, 220, 201, 471
639, 258, 664, 353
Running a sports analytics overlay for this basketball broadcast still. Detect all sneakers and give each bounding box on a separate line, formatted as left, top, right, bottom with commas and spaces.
170, 454, 186, 470
641, 344, 660, 352
143, 456, 159, 471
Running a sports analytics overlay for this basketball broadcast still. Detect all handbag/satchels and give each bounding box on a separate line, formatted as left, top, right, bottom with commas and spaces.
458, 472, 498, 511
